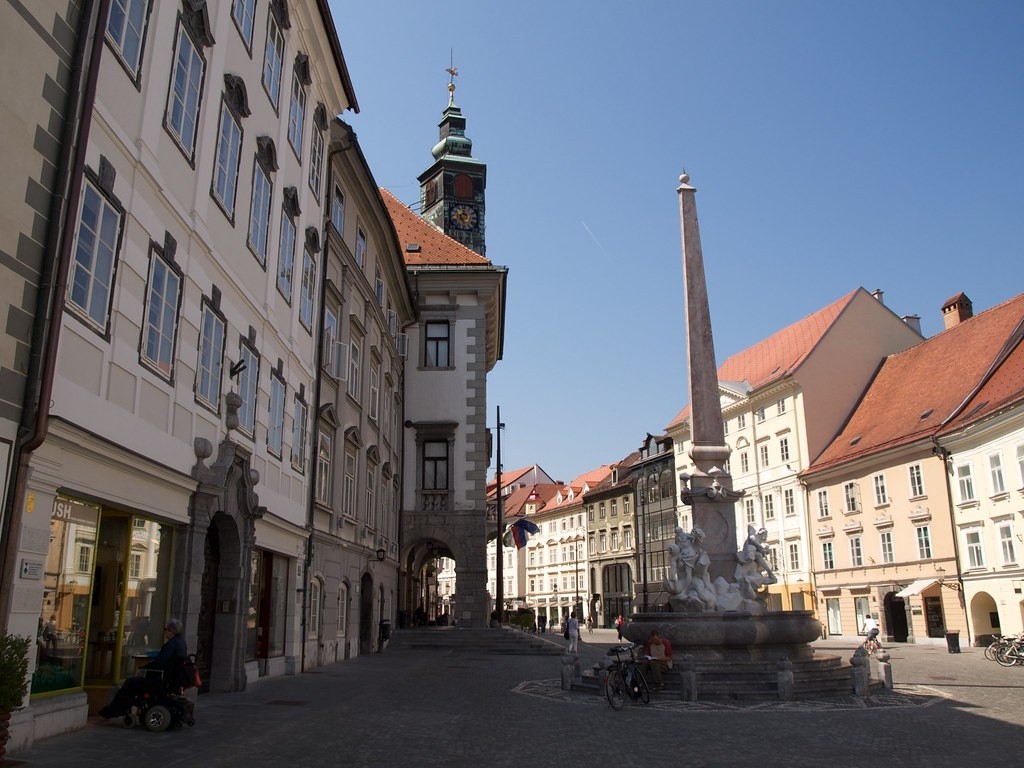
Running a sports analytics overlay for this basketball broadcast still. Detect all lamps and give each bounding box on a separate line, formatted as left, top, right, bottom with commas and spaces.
797, 579, 815, 596
432, 546, 441, 559
369, 548, 386, 562
427, 539, 433, 550
361, 527, 367, 538
336, 517, 345, 528
936, 566, 962, 590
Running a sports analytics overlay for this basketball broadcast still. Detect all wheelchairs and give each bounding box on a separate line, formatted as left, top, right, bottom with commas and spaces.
122, 652, 202, 734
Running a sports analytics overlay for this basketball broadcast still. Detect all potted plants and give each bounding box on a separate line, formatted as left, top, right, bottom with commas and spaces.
0, 627, 32, 763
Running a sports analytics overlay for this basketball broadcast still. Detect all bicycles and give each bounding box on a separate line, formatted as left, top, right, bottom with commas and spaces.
602, 640, 652, 711
983, 634, 1024, 667
859, 631, 882, 655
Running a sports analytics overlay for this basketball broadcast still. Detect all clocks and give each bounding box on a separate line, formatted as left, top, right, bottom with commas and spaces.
449, 205, 479, 230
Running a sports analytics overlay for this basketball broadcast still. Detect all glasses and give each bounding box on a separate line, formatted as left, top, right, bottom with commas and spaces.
163, 627, 168, 631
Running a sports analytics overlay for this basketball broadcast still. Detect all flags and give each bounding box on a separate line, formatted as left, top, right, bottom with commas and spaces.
504, 531, 516, 548
513, 527, 526, 549
511, 520, 541, 534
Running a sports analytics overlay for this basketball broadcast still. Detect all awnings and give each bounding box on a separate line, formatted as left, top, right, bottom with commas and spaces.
895, 580, 940, 598
530, 602, 576, 607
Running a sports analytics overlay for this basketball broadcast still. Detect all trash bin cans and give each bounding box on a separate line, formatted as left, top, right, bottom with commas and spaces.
944, 630, 961, 654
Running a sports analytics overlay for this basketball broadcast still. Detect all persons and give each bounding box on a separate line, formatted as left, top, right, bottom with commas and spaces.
861, 615, 880, 654
44, 614, 56, 648
99, 619, 188, 719
416, 604, 428, 625
438, 609, 450, 625
537, 612, 547, 633
549, 616, 554, 630
567, 613, 578, 653
749, 528, 777, 587
615, 615, 624, 640
670, 527, 699, 586
639, 630, 673, 692
587, 614, 594, 631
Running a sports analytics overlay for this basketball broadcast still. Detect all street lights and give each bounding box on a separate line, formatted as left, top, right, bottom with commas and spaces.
643, 472, 662, 612
574, 534, 586, 621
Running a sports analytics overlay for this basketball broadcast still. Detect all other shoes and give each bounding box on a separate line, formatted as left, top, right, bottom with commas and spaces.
653, 683, 664, 691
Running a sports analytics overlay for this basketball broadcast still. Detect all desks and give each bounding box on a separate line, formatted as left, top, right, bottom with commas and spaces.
88, 641, 116, 679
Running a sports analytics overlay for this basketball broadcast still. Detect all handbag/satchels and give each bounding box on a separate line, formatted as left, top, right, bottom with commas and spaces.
564, 619, 573, 640
181, 657, 202, 687
